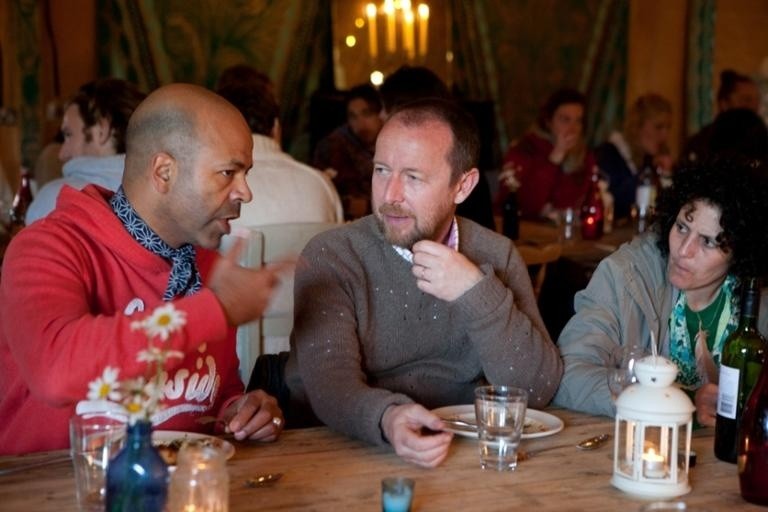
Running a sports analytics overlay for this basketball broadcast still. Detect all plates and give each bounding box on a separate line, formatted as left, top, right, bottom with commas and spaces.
428, 404, 564, 439
80, 428, 236, 470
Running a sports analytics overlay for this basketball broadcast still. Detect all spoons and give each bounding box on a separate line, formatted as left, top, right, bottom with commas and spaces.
514, 434, 610, 459
228, 471, 285, 487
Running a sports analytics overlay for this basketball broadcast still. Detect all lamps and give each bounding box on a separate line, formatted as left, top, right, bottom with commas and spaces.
610, 326, 694, 503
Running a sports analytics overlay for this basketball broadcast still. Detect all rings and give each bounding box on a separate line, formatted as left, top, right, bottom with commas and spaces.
273, 417, 281, 426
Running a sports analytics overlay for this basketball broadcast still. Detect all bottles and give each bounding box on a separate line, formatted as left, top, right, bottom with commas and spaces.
10, 161, 33, 231
502, 190, 520, 240
736, 368, 768, 503
631, 153, 657, 239
581, 165, 605, 240
104, 419, 169, 512
598, 180, 615, 235
709, 267, 768, 465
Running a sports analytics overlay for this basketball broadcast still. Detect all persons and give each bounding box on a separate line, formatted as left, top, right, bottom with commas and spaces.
555, 143, 768, 432
596, 91, 675, 222
215, 65, 344, 391
25, 77, 149, 227
283, 98, 565, 470
0, 82, 295, 469
374, 65, 496, 232
311, 83, 383, 224
718, 67, 762, 113
694, 107, 768, 168
33, 96, 64, 193
496, 87, 604, 347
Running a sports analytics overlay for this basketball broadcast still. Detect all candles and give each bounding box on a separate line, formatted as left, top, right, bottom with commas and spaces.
357, 0, 431, 65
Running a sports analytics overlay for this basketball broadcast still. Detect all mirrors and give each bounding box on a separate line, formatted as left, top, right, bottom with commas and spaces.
317, 0, 459, 95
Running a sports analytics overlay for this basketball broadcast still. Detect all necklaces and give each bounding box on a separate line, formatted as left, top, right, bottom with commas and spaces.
683, 287, 725, 385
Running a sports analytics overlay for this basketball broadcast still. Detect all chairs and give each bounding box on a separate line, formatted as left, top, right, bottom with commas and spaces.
510, 240, 564, 304
240, 219, 332, 387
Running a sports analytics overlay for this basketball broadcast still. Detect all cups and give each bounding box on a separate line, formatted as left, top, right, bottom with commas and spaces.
555, 207, 581, 243
606, 343, 652, 396
474, 384, 530, 471
378, 474, 416, 511
171, 440, 229, 512
68, 409, 126, 510
